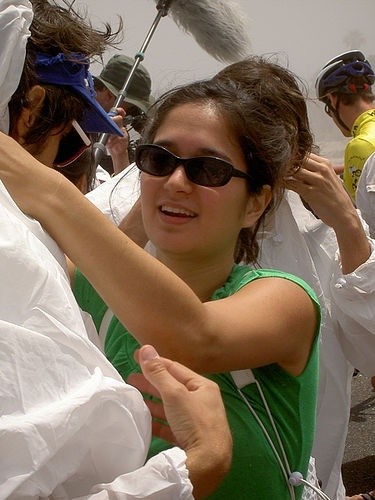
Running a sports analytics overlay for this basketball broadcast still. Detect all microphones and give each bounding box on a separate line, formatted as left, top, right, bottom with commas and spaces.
157, 0, 253, 63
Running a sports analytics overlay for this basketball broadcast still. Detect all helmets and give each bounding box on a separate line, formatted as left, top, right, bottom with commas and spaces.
314, 50, 375, 105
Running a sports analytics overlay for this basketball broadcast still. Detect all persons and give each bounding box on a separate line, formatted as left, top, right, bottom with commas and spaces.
1, 1, 234, 499
89, 54, 157, 187
314, 49, 375, 211
83, 56, 374, 500
1, 79, 323, 500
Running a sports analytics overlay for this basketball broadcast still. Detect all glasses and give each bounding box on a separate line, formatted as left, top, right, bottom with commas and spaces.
134, 143, 256, 187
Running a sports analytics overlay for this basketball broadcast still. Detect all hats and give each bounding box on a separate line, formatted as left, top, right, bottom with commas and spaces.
91, 54, 152, 118
30, 52, 124, 137
